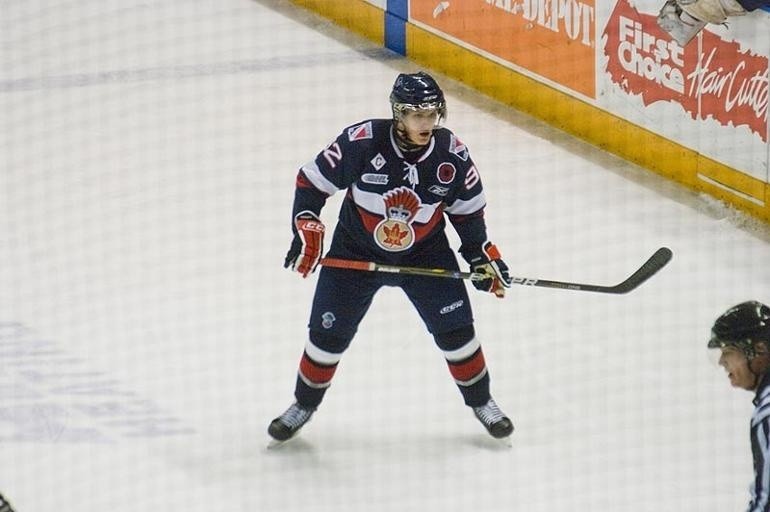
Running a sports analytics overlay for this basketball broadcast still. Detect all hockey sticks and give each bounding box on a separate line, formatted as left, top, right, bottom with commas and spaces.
317, 246, 672, 294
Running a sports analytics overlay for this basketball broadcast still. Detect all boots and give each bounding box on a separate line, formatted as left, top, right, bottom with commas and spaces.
267, 398, 316, 442
473, 396, 515, 440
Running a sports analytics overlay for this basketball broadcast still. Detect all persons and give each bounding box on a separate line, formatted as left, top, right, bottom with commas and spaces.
269, 72, 513, 441
706, 302, 766, 512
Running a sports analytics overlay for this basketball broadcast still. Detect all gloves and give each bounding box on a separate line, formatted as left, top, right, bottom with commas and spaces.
283, 219, 326, 278
457, 240, 512, 299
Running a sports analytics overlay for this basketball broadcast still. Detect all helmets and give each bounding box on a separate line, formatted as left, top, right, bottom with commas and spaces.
389, 71, 446, 108
707, 300, 770, 356
